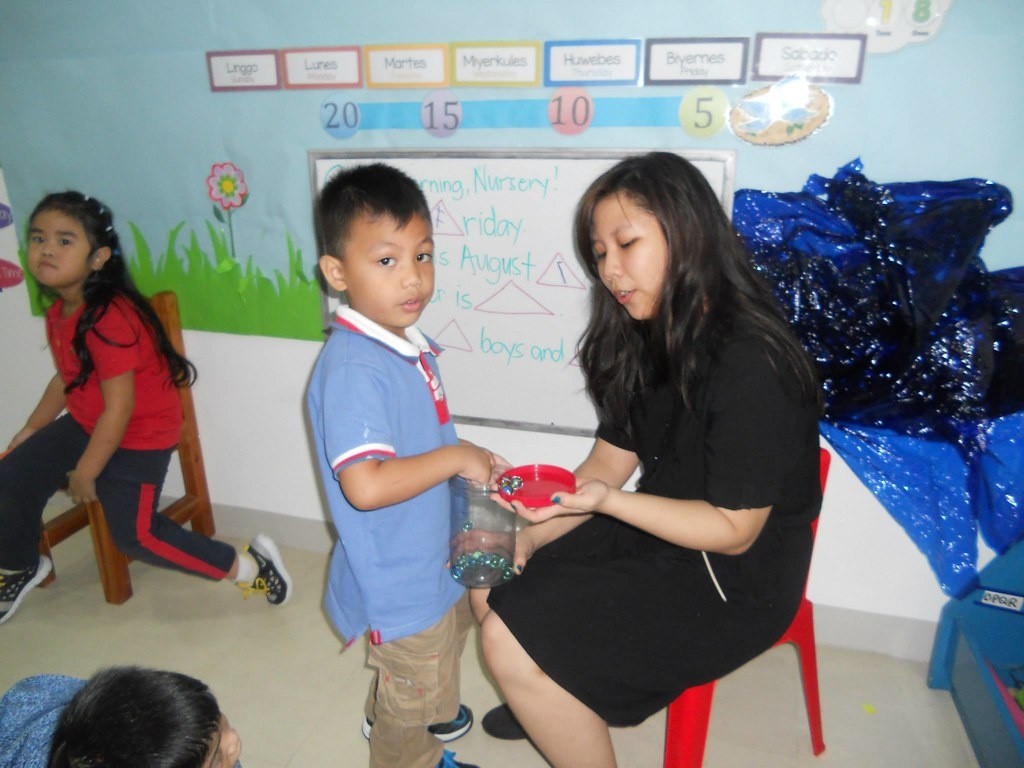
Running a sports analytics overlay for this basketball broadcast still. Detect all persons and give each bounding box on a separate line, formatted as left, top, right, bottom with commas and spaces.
1, 667, 241, 768
445, 152, 822, 768
0, 190, 295, 626
304, 162, 503, 767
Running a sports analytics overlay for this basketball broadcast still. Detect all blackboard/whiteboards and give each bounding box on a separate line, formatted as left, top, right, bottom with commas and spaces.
307, 149, 736, 438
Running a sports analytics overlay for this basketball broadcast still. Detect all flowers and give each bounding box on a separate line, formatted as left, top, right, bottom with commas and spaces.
207, 163, 250, 258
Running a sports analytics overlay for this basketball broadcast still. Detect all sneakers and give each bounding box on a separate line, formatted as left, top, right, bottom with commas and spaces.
362, 704, 473, 742
236, 533, 292, 607
0, 555, 52, 624
435, 749, 480, 768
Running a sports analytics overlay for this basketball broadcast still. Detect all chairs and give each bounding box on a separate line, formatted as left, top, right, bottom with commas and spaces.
36, 290, 214, 604
663, 446, 830, 768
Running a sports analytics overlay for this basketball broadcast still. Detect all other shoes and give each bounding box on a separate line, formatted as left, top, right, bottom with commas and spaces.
481, 702, 528, 740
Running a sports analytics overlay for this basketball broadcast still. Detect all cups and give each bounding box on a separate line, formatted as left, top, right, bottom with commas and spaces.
447, 475, 518, 588
